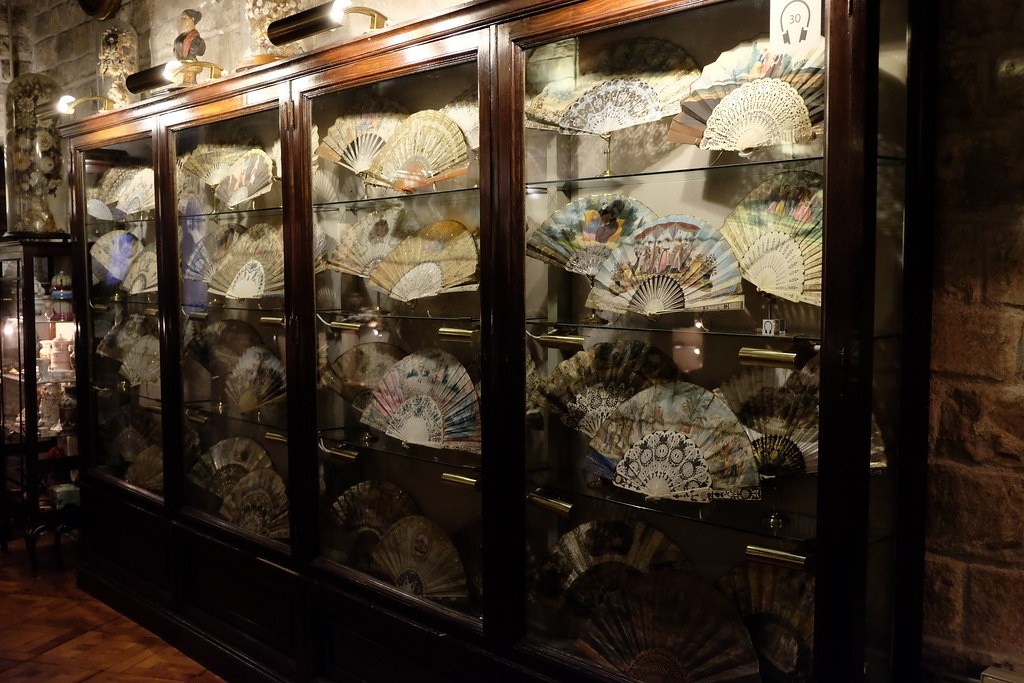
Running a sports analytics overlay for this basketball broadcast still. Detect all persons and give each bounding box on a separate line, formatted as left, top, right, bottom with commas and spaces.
173, 9, 206, 83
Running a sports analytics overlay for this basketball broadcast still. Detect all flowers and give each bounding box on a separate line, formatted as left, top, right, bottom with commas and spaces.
5, 76, 61, 198
245, 0, 306, 57
98, 23, 138, 76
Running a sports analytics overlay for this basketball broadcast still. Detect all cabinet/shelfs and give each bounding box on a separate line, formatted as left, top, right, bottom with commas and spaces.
0, 242, 96, 579
59, 1, 946, 683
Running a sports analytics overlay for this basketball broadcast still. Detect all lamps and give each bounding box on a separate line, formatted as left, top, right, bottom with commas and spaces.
267, 0, 388, 46
34, 94, 118, 122
126, 61, 224, 94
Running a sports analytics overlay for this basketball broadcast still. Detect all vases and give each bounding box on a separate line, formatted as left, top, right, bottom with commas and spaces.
16, 190, 56, 232
106, 81, 129, 108
